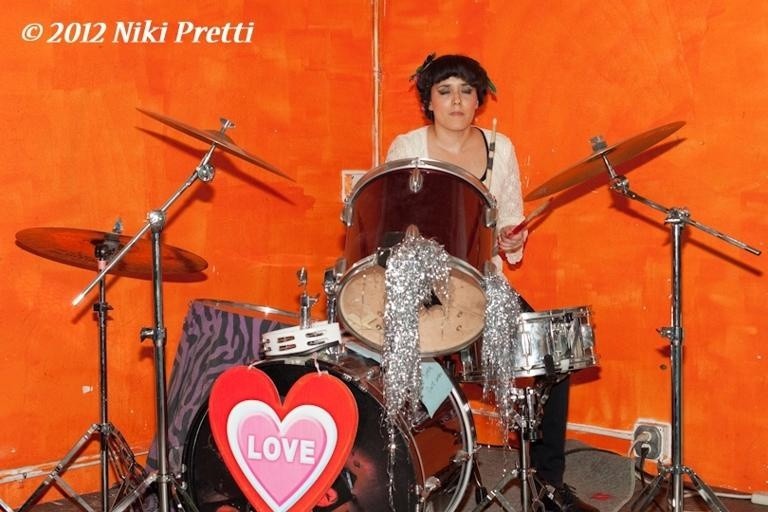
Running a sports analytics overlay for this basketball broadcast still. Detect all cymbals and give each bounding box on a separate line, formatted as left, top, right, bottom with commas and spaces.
523, 120, 686, 200
15, 227, 208, 275
136, 106, 293, 181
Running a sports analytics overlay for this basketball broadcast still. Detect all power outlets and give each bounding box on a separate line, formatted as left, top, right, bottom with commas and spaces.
634, 425, 661, 460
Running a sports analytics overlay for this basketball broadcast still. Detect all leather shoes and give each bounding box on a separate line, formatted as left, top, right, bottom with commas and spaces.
541, 483, 599, 512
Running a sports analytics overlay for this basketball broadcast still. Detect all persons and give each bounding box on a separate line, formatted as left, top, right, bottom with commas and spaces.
386, 51, 602, 511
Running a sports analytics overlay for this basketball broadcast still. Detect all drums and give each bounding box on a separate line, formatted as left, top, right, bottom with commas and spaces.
341, 157, 498, 358
183, 332, 477, 512
147, 298, 326, 479
463, 306, 601, 383
